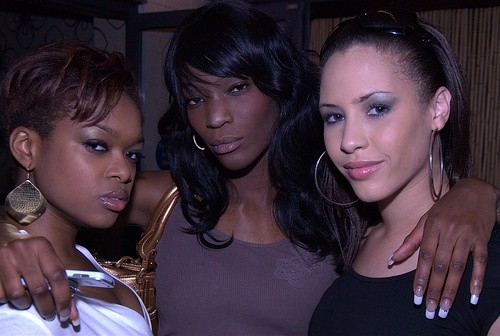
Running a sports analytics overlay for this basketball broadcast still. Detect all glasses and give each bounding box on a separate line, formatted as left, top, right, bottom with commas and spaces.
317, 7, 431, 62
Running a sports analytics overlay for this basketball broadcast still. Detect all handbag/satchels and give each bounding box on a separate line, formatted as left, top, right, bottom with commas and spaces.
93, 185, 182, 336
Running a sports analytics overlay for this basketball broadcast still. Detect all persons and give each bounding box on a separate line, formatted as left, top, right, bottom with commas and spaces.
1, 41, 155, 336
308, 16, 500, 336
0, 1, 500, 327
